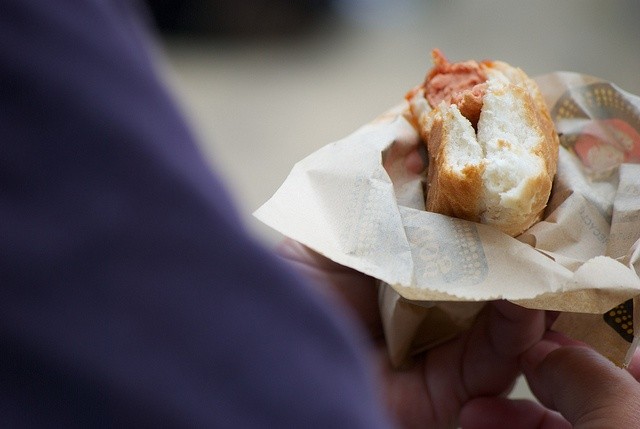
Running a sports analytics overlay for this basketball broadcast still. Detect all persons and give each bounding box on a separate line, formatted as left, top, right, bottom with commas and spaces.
0, 0, 639, 428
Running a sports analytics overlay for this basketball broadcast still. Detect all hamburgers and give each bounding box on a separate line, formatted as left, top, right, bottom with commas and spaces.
401, 48, 561, 237
